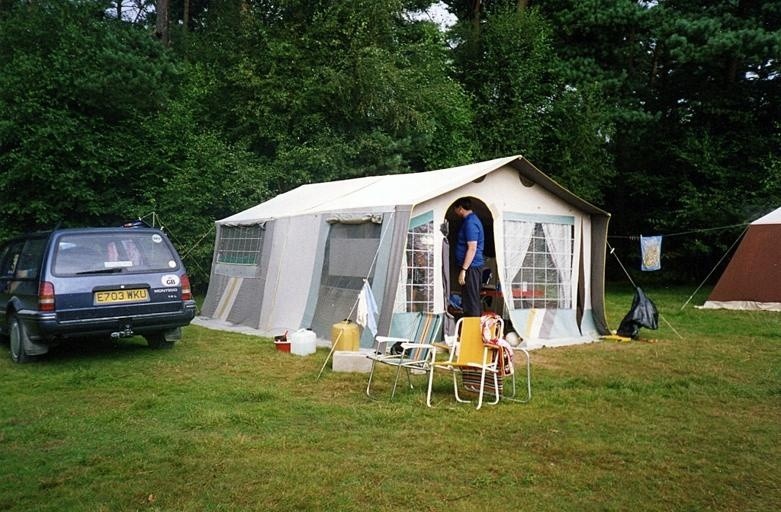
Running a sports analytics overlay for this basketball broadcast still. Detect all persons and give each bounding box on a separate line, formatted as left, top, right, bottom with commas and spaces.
454, 198, 485, 318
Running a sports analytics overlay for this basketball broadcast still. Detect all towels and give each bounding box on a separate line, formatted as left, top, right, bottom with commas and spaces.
639, 234, 664, 272
357, 279, 381, 338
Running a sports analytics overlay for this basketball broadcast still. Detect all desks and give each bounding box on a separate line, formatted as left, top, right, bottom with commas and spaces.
480, 288, 547, 318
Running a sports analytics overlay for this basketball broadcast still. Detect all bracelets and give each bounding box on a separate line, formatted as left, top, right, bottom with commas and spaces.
461, 267, 467, 272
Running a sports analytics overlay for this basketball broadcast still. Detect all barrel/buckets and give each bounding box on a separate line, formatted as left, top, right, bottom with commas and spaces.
331, 319, 360, 351
290, 327, 316, 356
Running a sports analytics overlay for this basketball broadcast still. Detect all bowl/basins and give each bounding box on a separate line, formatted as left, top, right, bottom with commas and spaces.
274, 341, 290, 353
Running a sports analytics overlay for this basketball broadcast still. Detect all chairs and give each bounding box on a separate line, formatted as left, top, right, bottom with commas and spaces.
366, 310, 533, 411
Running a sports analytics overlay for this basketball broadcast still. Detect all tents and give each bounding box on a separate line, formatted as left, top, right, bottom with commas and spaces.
188, 152, 613, 365
693, 206, 781, 312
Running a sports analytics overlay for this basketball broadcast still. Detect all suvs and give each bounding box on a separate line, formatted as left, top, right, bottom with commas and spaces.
3, 230, 197, 362
